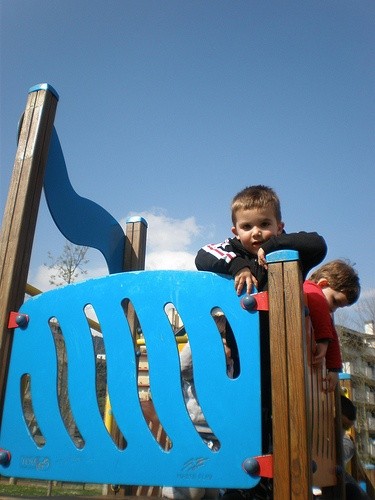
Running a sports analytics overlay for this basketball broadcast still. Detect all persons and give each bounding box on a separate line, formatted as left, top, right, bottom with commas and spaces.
302, 260, 361, 391
195, 185, 327, 500
162, 329, 234, 500
321, 397, 357, 466
